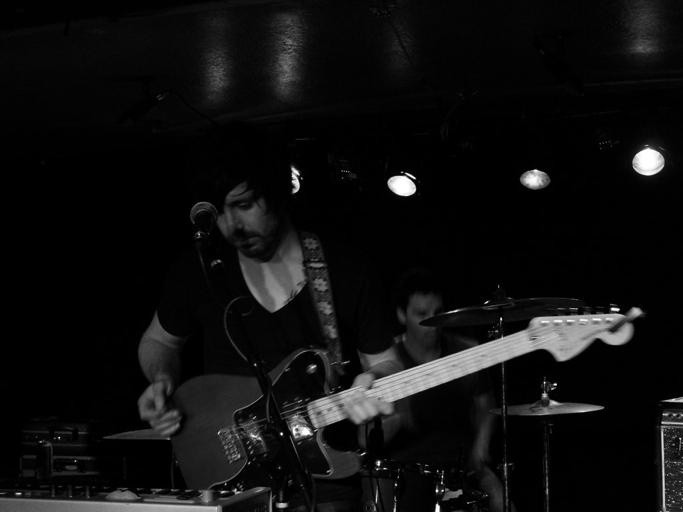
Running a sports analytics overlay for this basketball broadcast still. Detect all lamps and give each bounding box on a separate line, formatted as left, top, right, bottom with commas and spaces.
384, 167, 422, 199
630, 140, 668, 179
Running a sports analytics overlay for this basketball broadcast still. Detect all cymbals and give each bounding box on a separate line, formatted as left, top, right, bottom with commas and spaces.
419, 297, 580, 326
488, 399, 605, 417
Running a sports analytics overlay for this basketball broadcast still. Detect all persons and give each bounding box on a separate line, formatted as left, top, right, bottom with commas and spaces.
137, 167, 390, 512
380, 277, 497, 512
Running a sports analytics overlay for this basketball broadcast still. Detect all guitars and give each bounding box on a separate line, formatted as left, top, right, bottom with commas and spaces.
171, 305, 645, 489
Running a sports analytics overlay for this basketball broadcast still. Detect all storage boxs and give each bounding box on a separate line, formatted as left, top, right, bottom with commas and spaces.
654, 397, 683, 511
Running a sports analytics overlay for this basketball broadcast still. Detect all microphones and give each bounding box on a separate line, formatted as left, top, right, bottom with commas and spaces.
190, 202, 218, 248
